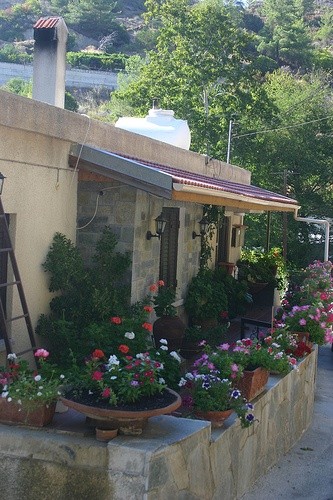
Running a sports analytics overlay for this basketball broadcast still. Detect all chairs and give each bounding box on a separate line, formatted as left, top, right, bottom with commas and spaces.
151, 315, 200, 363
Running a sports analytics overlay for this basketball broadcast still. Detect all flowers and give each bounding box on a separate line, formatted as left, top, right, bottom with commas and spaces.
0, 260, 333, 428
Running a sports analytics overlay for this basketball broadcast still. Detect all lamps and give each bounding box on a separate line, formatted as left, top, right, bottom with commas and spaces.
146, 212, 167, 240
192, 216, 209, 240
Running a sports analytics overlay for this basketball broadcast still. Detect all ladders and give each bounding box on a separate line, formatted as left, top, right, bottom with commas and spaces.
0, 199, 42, 373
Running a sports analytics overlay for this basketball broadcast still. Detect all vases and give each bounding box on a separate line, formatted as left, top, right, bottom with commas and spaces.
0, 396, 59, 427
188, 406, 234, 427
228, 364, 269, 404
286, 330, 310, 351
57, 384, 182, 441
